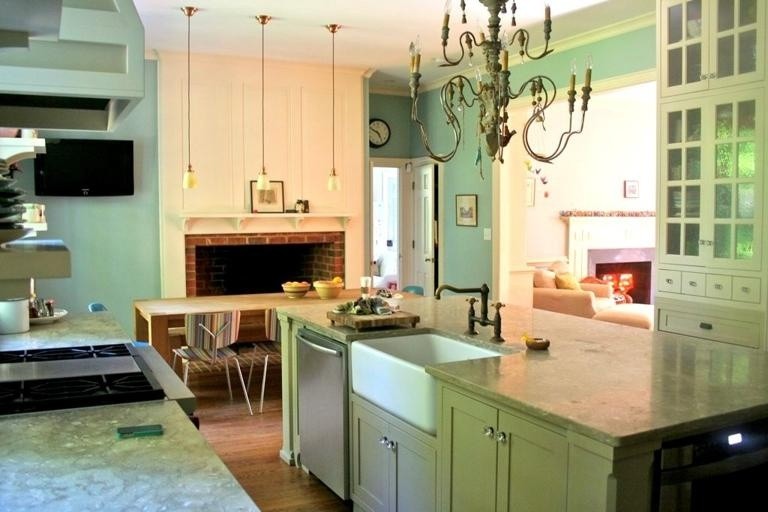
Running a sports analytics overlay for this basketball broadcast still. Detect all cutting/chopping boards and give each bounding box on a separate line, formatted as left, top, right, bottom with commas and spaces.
324, 308, 420, 331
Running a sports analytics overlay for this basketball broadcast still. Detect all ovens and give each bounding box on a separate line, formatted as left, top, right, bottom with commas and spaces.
293, 324, 356, 503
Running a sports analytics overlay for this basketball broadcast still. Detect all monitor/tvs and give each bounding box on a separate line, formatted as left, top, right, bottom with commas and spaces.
34, 138, 135, 197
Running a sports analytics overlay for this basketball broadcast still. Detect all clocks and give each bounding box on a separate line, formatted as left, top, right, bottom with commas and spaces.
368, 116, 391, 149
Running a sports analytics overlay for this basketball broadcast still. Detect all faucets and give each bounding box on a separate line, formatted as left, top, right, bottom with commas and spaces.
433, 279, 505, 343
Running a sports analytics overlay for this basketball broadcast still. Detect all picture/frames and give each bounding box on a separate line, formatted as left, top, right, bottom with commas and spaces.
456, 193, 478, 228
250, 179, 285, 214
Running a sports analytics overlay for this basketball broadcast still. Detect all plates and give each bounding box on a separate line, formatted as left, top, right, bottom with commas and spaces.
371, 292, 404, 304
29, 308, 67, 325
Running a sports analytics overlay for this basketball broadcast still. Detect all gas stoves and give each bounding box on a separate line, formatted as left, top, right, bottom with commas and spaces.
0, 343, 165, 412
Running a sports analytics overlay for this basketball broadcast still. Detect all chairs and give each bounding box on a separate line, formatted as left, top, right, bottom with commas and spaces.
527, 259, 615, 318
171, 309, 255, 416
242, 308, 281, 414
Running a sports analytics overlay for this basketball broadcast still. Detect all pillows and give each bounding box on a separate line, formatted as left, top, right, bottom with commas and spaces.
533, 269, 582, 289
589, 311, 651, 330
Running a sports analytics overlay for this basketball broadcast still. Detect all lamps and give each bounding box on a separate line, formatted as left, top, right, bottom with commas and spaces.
180, 6, 199, 193
322, 23, 341, 195
253, 14, 272, 191
408, 1, 592, 164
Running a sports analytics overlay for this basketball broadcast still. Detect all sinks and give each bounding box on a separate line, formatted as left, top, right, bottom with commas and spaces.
344, 324, 504, 436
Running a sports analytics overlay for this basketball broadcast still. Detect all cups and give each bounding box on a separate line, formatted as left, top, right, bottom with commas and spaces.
0, 297, 30, 334
359, 275, 372, 300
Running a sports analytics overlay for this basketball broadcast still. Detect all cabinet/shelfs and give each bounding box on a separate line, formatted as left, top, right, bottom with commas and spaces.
660, 1, 768, 349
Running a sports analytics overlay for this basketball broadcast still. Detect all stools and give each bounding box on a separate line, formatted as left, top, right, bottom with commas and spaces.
611, 302, 654, 330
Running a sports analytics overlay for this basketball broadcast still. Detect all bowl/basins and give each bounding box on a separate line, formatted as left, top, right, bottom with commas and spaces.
525, 337, 550, 350
312, 280, 346, 300
281, 281, 313, 300
